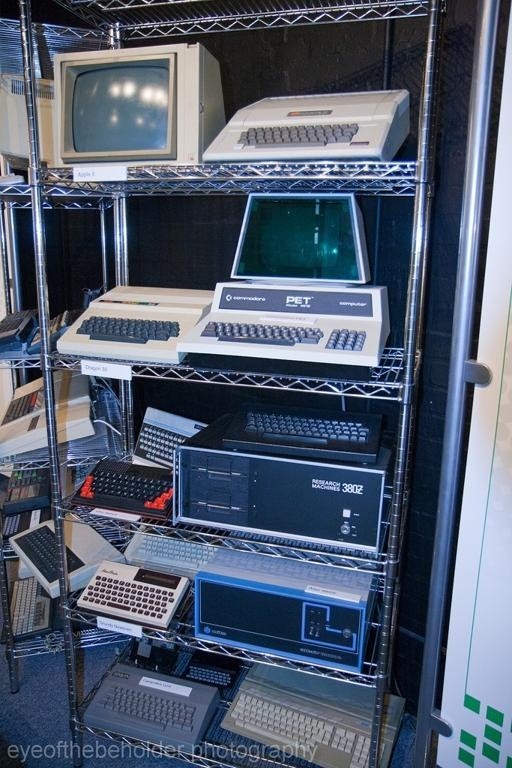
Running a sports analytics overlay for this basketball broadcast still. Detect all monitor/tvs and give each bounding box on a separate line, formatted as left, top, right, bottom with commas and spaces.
53, 43, 227, 168
230, 191, 370, 286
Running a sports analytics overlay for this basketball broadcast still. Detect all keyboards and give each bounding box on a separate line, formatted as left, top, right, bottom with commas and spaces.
1, 315, 407, 768
238, 121, 358, 146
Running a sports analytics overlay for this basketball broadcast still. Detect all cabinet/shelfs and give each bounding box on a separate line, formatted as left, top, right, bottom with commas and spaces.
18, 0, 447, 768
0, 18, 136, 693
